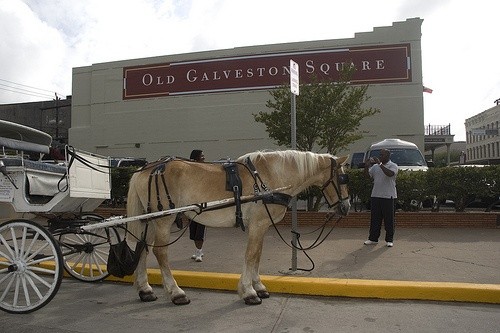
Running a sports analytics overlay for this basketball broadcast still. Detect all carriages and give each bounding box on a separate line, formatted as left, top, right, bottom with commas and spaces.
0, 119, 353, 315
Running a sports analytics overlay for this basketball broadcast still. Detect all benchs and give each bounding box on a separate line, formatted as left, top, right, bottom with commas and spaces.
0, 120, 70, 175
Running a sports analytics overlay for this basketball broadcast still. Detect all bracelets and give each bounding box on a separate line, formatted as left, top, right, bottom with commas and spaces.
378, 162, 382, 167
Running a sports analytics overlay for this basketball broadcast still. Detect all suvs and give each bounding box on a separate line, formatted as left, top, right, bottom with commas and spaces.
109, 156, 149, 170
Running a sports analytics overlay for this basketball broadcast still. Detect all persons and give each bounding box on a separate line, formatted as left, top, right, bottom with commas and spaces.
189, 149, 205, 262
364, 148, 398, 247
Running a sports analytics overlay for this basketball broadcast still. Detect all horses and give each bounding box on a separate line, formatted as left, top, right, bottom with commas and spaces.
124, 149, 350, 305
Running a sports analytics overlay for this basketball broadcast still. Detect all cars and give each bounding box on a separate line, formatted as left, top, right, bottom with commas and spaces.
436, 157, 500, 208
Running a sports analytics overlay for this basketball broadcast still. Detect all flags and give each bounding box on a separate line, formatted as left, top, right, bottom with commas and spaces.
423, 87, 432, 93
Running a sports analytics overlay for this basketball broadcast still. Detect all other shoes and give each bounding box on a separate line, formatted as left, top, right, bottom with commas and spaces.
386, 242, 393, 246
191, 249, 204, 261
364, 240, 379, 245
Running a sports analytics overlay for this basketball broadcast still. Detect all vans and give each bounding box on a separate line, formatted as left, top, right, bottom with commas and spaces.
358, 138, 429, 173
